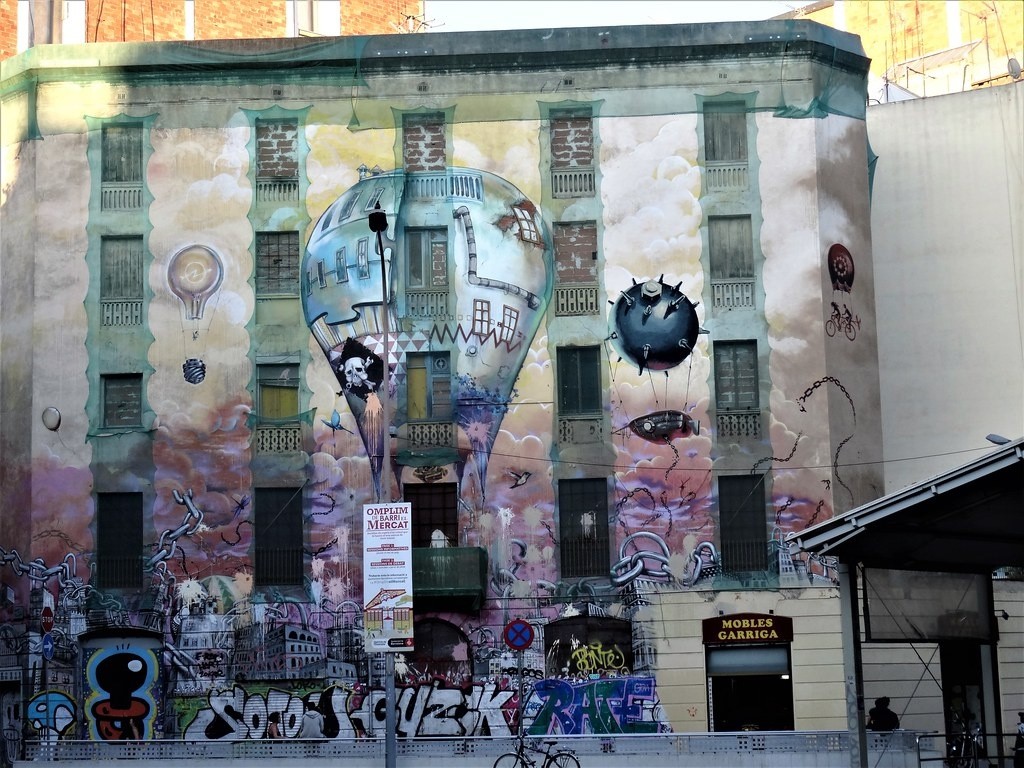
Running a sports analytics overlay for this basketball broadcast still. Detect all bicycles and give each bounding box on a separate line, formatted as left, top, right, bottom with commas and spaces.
493, 729, 580, 768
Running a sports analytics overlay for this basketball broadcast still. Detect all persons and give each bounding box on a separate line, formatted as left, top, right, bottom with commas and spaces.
1010, 712, 1024, 768
866, 697, 900, 736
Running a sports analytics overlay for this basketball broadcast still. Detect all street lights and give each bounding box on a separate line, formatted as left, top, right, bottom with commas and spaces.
369, 196, 428, 768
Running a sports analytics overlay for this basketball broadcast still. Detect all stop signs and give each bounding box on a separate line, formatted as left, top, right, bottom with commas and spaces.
42, 606, 54, 634
